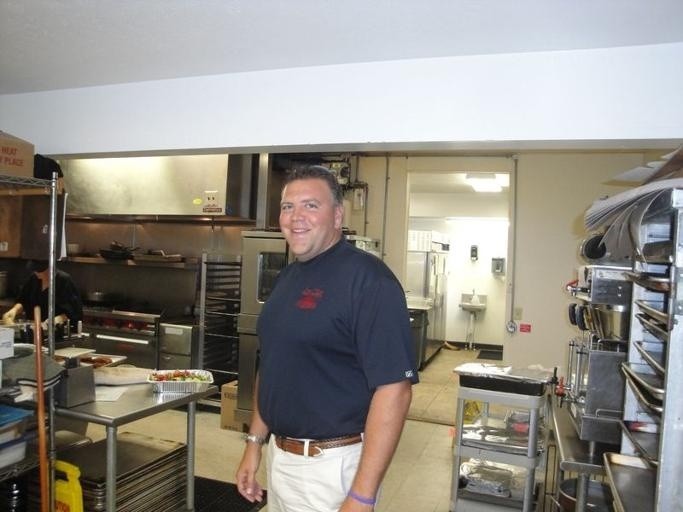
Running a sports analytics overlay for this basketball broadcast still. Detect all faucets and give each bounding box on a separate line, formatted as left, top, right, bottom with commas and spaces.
472, 288, 475, 296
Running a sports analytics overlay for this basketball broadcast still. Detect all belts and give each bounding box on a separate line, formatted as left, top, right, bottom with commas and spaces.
274, 432, 364, 458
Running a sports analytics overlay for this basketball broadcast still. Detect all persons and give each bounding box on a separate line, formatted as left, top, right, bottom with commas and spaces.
2, 259, 83, 337
234, 165, 420, 512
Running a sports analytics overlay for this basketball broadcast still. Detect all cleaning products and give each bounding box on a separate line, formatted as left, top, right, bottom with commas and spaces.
50, 461, 83, 512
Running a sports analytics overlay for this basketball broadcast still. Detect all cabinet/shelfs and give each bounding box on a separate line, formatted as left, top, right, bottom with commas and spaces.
448, 385, 545, 511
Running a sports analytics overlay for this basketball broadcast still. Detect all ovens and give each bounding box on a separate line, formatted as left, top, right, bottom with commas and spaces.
231, 230, 381, 425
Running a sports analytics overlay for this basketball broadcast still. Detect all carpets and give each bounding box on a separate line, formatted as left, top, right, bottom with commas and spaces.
192, 474, 268, 511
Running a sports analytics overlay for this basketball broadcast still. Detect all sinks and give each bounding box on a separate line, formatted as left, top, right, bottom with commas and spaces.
460, 303, 485, 311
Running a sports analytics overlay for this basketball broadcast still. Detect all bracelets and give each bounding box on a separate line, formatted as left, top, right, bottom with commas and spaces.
347, 490, 376, 505
244, 433, 264, 445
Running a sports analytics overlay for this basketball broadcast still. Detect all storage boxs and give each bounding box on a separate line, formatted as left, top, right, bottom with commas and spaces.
219, 378, 241, 432
